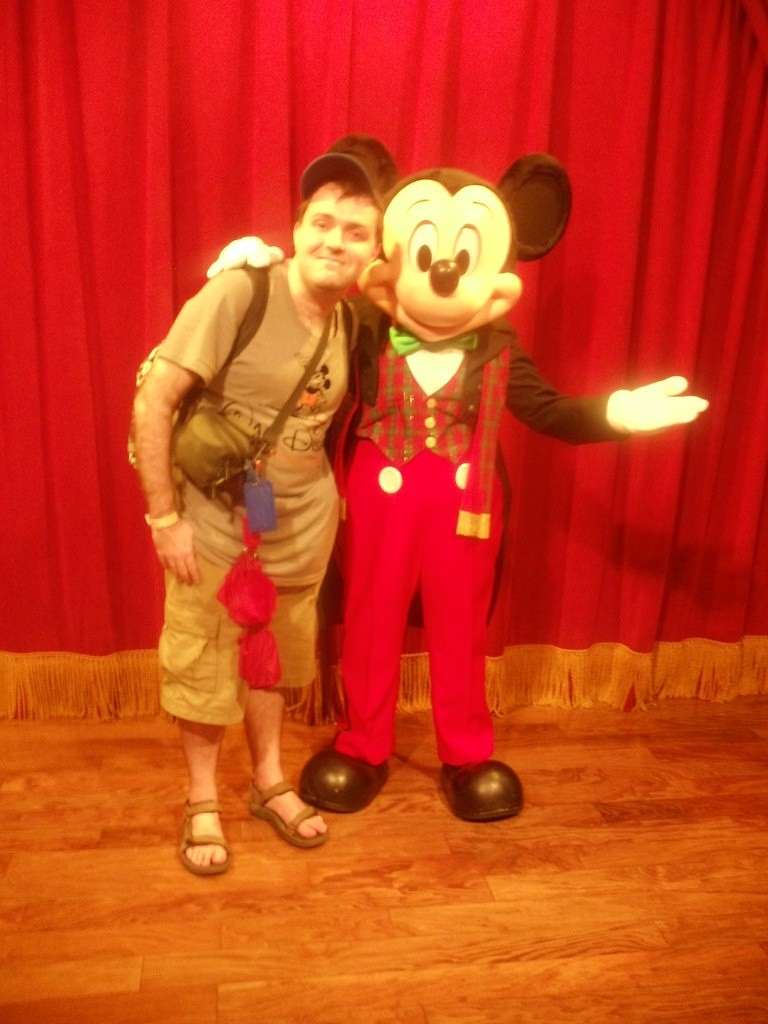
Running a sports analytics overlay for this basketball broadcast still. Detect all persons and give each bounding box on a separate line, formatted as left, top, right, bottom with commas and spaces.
133, 175, 387, 873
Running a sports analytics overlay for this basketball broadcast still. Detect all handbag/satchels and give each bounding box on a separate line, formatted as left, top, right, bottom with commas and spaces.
171, 408, 261, 499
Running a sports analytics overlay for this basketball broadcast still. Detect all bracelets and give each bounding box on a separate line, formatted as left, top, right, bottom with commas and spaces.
144, 511, 180, 529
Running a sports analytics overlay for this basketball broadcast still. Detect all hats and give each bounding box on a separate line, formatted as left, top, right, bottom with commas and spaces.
300, 134, 398, 209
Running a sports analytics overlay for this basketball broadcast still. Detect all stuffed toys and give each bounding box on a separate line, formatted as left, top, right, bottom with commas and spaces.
203, 129, 712, 822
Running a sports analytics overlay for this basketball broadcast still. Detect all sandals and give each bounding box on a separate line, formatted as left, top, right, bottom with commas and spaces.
251, 780, 329, 848
179, 798, 231, 874
